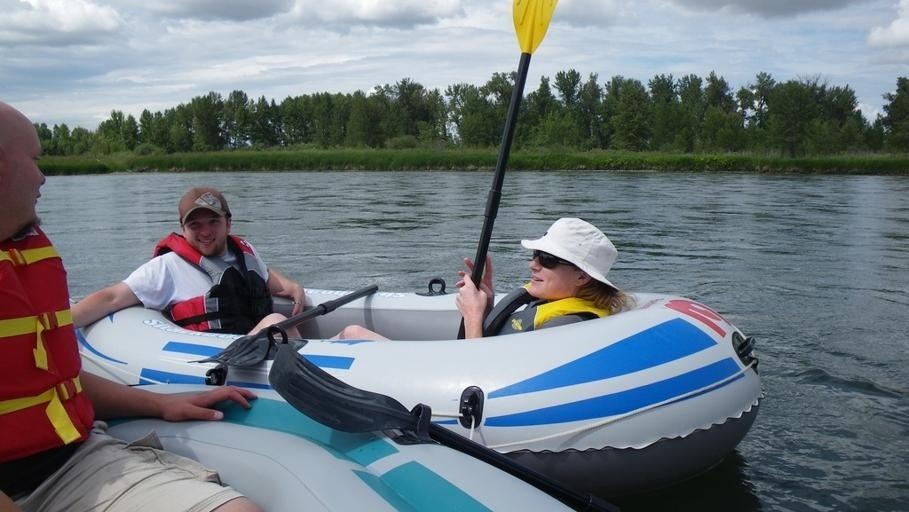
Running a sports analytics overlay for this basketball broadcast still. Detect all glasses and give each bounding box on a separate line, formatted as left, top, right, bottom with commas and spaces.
534, 250, 575, 268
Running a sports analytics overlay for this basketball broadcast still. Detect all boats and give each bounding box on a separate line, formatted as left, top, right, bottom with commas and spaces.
94, 383, 623, 512
71, 286, 765, 502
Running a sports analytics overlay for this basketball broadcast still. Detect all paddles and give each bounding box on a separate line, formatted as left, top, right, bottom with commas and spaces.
190, 280, 379, 371
270, 346, 652, 512
457, 1, 560, 350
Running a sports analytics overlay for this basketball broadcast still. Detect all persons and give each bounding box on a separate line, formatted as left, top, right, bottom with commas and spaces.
329, 216, 639, 341
1, 100, 266, 512
71, 188, 305, 340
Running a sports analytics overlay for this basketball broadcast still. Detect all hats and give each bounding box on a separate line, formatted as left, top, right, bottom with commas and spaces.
179, 188, 231, 224
521, 218, 621, 292
